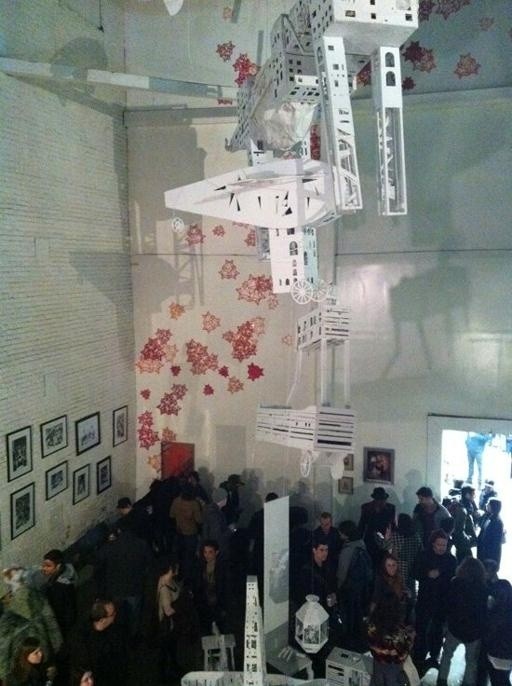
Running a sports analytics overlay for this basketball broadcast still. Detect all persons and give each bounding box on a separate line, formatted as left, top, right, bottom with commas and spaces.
1, 432, 512, 686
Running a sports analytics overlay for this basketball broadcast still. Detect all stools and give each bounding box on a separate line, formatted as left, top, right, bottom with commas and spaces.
202, 634, 236, 671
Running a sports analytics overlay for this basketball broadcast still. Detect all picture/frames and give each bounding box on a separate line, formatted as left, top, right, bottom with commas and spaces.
338, 454, 354, 495
362, 446, 396, 485
6, 405, 139, 542
